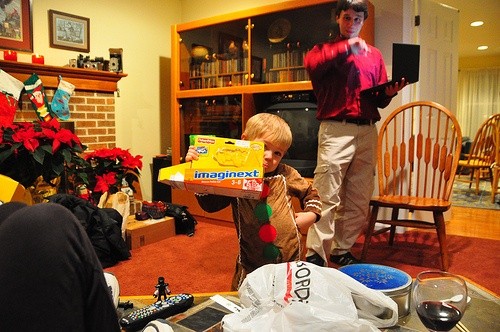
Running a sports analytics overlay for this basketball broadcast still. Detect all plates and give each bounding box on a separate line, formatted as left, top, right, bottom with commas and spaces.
267, 18, 291, 43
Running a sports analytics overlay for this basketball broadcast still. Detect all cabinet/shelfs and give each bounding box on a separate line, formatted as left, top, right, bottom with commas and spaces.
171, 0, 375, 235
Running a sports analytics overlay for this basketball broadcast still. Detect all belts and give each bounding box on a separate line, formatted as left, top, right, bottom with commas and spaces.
324, 115, 377, 125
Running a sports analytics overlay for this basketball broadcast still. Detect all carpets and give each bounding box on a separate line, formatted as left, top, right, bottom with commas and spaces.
325, 230, 500, 298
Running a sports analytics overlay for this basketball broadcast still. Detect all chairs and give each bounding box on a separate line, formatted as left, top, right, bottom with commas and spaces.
359, 100, 500, 274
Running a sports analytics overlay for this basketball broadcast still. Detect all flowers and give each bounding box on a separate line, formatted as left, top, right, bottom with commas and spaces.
0, 116, 143, 206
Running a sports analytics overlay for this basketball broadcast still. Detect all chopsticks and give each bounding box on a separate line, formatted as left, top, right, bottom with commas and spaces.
456, 321, 470, 332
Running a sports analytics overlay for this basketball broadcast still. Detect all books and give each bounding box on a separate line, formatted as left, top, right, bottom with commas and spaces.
189, 50, 311, 87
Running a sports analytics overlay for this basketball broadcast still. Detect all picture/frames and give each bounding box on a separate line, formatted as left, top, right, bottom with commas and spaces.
48, 9, 90, 53
0, 0, 33, 53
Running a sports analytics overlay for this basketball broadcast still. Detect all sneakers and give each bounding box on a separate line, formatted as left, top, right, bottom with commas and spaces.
305, 252, 325, 267
330, 252, 361, 266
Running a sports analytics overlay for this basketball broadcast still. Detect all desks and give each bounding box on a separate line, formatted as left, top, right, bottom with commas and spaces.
118, 275, 500, 332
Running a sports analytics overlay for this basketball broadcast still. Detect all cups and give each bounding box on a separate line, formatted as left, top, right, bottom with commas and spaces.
413, 271, 468, 332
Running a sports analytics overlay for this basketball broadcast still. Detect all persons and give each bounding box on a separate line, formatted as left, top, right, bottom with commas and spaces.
0, 201, 173, 332
302, 0, 411, 267
186, 113, 321, 291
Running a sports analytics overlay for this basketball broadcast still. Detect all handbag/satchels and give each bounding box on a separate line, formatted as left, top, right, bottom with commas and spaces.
164, 203, 198, 236
220, 260, 399, 331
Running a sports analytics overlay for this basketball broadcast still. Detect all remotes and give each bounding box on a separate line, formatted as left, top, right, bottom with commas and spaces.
119, 292, 194, 332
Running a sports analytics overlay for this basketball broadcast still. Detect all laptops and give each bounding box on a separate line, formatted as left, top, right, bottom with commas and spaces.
360, 43, 420, 95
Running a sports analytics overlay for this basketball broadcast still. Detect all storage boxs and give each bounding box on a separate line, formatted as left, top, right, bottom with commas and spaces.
158, 135, 265, 200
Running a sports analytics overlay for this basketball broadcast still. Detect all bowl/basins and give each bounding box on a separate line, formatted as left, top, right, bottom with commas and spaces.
338, 264, 413, 319
